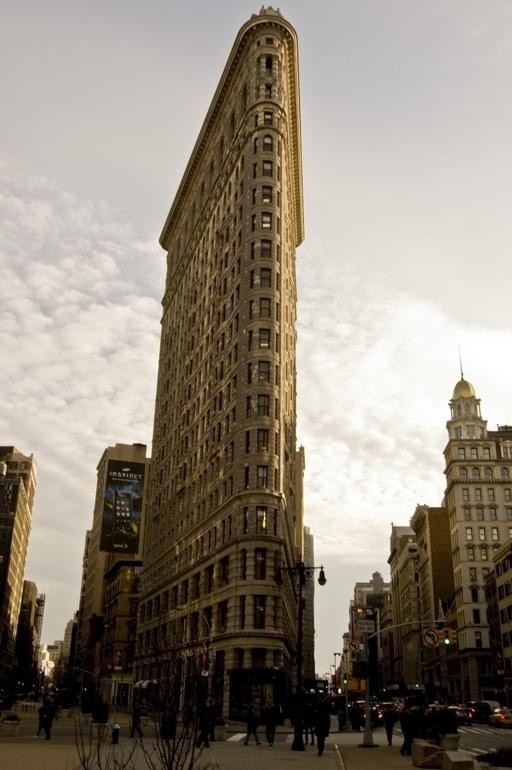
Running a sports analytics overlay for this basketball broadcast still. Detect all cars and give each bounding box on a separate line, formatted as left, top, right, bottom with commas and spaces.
348, 699, 512, 728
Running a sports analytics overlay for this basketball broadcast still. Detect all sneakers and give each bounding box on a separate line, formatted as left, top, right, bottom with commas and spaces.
129, 734, 144, 738
244, 742, 273, 746
305, 742, 314, 745
45, 737, 50, 740
196, 740, 216, 748
400, 750, 411, 756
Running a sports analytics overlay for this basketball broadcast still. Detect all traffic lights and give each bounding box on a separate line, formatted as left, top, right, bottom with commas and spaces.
442, 628, 451, 646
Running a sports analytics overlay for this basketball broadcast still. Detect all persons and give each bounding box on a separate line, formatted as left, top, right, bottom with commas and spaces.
31, 701, 58, 739
264, 703, 278, 746
208, 699, 217, 743
39, 703, 52, 741
304, 693, 459, 757
244, 705, 263, 747
127, 708, 145, 739
194, 698, 215, 749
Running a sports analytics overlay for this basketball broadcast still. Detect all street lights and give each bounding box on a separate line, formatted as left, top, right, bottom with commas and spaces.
357, 607, 377, 632
274, 554, 326, 751
